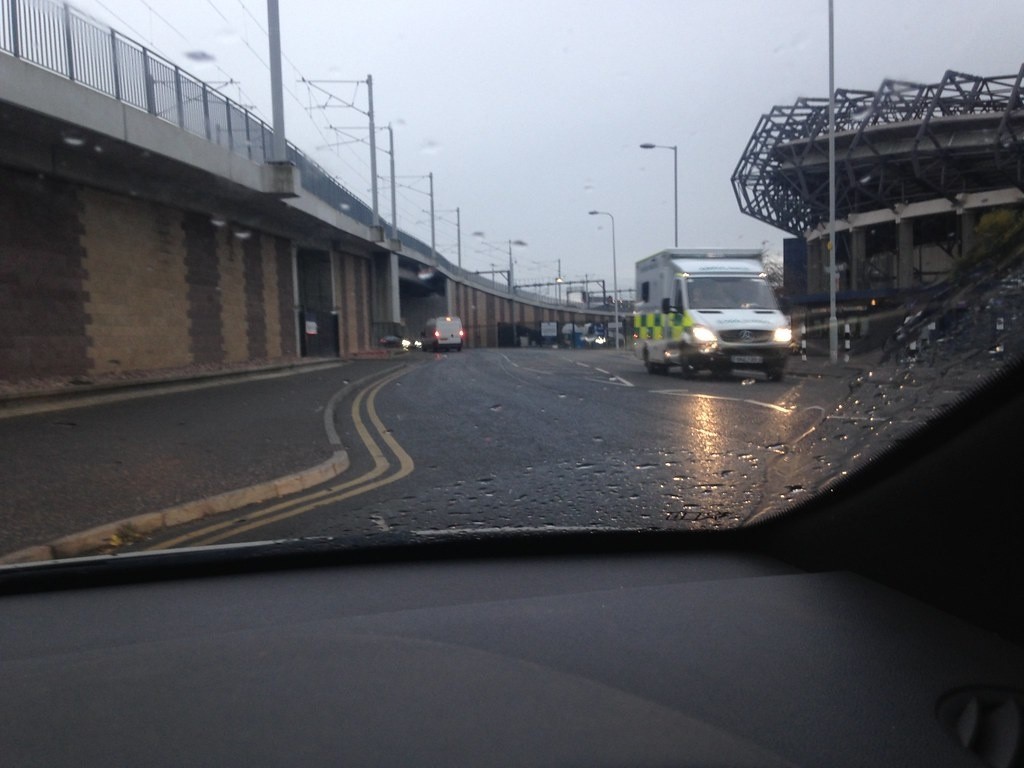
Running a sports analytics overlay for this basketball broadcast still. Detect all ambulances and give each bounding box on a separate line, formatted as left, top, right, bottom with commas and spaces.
634, 247, 793, 380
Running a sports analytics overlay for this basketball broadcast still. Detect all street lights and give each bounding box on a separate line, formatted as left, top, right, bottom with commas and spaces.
508, 237, 528, 345
589, 211, 620, 349
639, 142, 679, 248
458, 231, 484, 316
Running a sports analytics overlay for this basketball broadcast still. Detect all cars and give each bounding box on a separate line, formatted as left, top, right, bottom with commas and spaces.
382, 333, 421, 349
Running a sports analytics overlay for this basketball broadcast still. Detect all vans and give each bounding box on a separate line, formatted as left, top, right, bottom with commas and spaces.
420, 317, 463, 350
